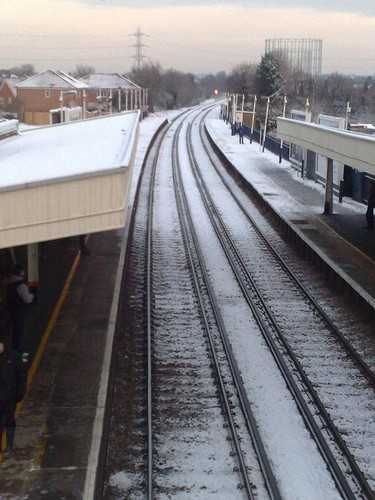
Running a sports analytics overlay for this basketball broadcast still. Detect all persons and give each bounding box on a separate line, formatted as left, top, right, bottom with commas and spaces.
3, 265, 36, 363
237, 124, 245, 144
79, 234, 93, 257
362, 181, 375, 229
0, 334, 29, 456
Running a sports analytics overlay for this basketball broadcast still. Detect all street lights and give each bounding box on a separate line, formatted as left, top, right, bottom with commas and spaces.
228, 94, 237, 135
58, 91, 65, 123
337, 102, 351, 203
301, 98, 310, 178
250, 95, 258, 144
118, 88, 149, 122
262, 98, 271, 152
108, 89, 113, 115
82, 89, 88, 120
96, 89, 102, 116
279, 95, 288, 164
241, 94, 245, 127
